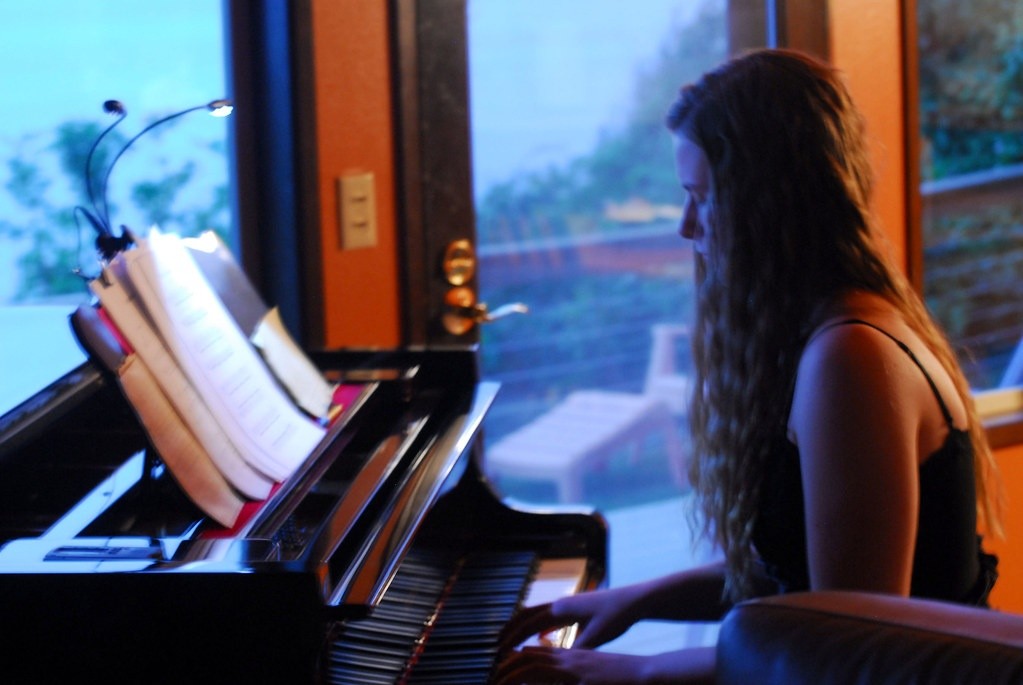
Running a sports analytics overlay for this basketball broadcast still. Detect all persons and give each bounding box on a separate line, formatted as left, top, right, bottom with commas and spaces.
490, 50, 1004, 685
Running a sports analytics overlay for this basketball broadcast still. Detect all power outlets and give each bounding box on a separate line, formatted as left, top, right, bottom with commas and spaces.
335, 169, 378, 252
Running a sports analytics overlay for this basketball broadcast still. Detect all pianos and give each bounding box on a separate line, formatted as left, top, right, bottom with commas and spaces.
0, 340, 611, 685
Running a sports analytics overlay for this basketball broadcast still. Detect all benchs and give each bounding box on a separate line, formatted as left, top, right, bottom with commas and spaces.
486, 389, 690, 506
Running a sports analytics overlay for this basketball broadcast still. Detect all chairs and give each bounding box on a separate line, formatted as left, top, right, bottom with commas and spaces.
630, 323, 693, 466
711, 383, 1023, 685
